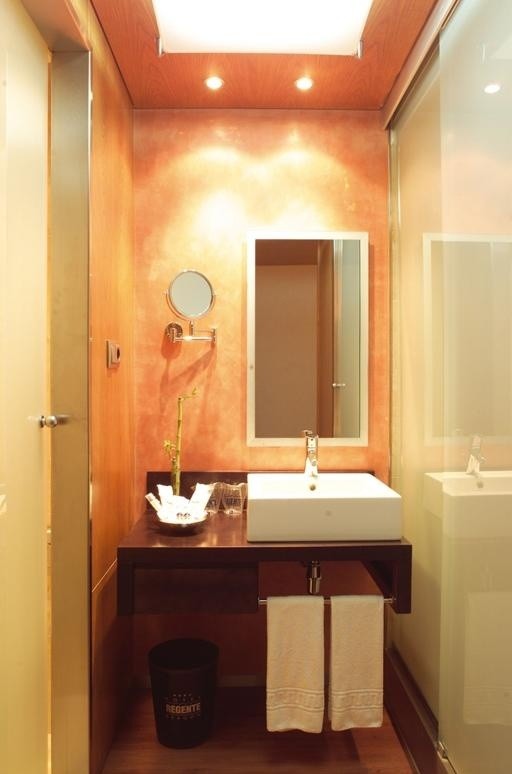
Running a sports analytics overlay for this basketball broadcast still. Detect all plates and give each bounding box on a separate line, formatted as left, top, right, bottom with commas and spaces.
157, 511, 208, 531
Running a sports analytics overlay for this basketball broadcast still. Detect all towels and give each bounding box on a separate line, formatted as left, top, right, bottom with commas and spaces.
462, 591, 512, 728
265, 595, 385, 734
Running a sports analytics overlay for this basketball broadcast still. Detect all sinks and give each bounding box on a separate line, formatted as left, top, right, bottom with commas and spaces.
245, 473, 402, 543
424, 470, 512, 538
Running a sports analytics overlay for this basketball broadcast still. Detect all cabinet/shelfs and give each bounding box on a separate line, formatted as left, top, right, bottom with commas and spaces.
116, 546, 273, 616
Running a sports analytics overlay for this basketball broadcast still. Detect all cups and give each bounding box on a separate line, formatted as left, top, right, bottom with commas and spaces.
192, 482, 247, 516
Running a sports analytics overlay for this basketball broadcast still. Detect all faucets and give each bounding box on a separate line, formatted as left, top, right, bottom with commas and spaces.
302, 430, 319, 475
467, 436, 487, 474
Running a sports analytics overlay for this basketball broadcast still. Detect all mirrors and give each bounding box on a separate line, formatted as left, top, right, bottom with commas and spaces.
248, 232, 369, 448
423, 231, 510, 449
